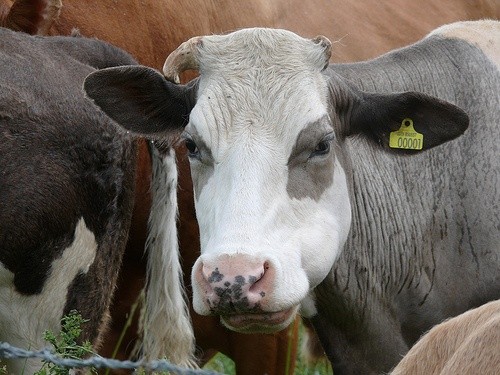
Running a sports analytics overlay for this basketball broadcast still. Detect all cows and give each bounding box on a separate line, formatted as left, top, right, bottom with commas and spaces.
0, 0, 500, 374
